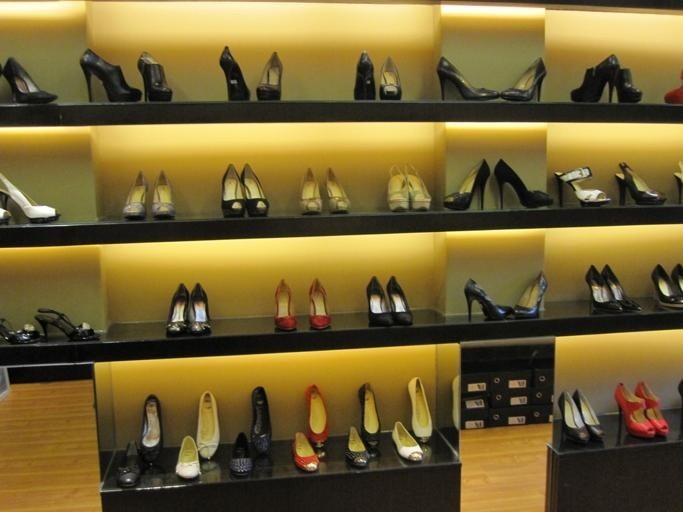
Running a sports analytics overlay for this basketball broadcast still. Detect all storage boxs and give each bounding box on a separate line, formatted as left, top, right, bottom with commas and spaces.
459, 346, 552, 431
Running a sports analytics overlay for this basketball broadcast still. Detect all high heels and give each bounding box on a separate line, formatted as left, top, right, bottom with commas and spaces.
80, 48, 172, 102
220, 46, 281, 100
2, 58, 56, 103
615, 163, 667, 205
0, 308, 100, 344
571, 55, 641, 102
354, 50, 401, 100
495, 159, 553, 209
615, 382, 669, 438
437, 56, 546, 102
443, 159, 490, 210
465, 272, 547, 321
559, 389, 605, 444
555, 166, 611, 207
0, 173, 60, 223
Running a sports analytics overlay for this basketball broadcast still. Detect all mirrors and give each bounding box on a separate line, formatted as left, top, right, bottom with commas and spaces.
457, 339, 556, 511
0, 359, 100, 510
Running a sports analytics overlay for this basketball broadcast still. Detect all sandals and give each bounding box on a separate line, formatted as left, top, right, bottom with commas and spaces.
222, 163, 269, 217
387, 163, 431, 212
123, 170, 175, 220
300, 168, 350, 215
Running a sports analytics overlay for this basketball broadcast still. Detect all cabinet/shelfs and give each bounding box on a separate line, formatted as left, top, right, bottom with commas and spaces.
0, 100, 682, 512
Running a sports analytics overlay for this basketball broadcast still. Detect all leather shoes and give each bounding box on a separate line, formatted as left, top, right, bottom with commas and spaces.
167, 283, 211, 336
251, 386, 272, 454
367, 276, 413, 326
345, 426, 368, 467
391, 421, 423, 461
137, 394, 163, 464
306, 383, 329, 447
228, 432, 252, 477
407, 377, 433, 443
292, 432, 319, 473
359, 383, 381, 448
274, 279, 297, 330
309, 278, 331, 329
196, 391, 220, 461
586, 264, 642, 312
652, 264, 683, 308
116, 441, 140, 487
176, 436, 200, 479
